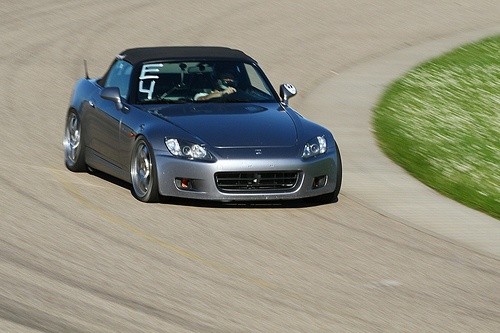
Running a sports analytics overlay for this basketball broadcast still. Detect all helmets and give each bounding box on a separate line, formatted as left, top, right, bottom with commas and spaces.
214, 63, 240, 89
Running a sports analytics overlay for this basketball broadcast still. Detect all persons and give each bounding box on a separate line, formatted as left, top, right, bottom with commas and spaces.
193, 60, 241, 101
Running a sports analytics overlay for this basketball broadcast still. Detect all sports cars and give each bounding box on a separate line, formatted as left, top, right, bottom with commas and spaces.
63, 45, 342, 207
